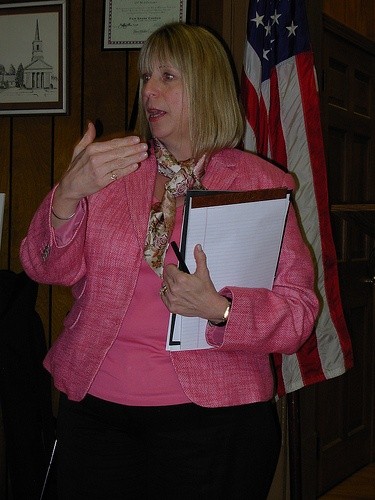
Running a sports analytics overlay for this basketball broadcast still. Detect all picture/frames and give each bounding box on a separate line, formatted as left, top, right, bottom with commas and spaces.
0, 0, 67, 116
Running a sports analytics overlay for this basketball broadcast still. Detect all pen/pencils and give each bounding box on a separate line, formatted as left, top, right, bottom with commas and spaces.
170, 241, 190, 275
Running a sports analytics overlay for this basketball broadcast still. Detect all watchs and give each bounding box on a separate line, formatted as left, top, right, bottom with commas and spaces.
208, 295, 232, 327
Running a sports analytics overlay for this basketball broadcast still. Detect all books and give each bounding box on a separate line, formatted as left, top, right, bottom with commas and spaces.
165, 187, 292, 351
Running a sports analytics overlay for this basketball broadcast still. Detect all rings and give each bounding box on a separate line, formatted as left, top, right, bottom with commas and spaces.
111, 172, 118, 181
159, 285, 168, 296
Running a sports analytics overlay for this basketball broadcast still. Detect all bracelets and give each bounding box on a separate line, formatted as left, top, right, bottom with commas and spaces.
51, 206, 75, 220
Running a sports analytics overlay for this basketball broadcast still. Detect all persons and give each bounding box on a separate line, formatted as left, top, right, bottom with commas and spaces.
20, 23, 319, 500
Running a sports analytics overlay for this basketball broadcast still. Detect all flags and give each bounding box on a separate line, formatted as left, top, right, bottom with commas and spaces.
239, 0, 354, 402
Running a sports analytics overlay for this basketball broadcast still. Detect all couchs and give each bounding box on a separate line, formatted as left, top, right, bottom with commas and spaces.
1, 270, 57, 499
